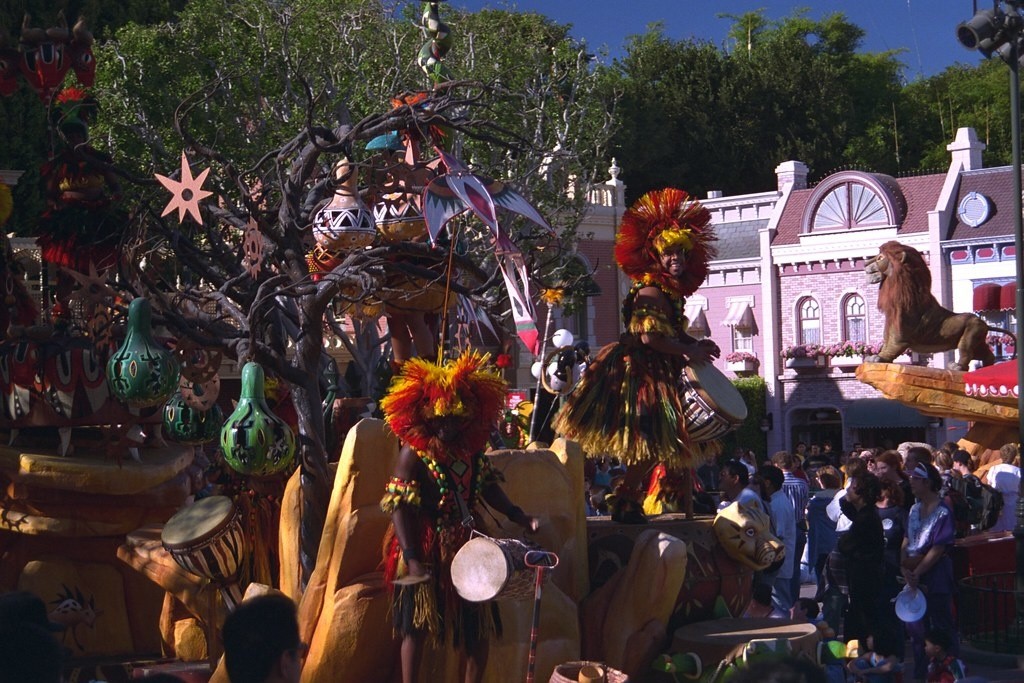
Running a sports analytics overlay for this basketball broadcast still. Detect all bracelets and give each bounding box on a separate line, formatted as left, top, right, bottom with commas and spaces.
402, 549, 416, 563
508, 506, 523, 520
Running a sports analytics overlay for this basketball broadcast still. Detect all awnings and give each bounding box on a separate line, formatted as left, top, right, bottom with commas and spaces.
845, 399, 928, 428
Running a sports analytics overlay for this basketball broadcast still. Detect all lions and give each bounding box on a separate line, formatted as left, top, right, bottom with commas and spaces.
864, 240, 1017, 371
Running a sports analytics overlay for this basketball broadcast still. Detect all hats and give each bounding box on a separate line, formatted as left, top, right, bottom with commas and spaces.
902, 462, 929, 479
895, 584, 926, 622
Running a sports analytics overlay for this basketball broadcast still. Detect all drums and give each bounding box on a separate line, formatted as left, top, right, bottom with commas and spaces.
159, 494, 249, 608
450, 535, 552, 602
636, 360, 746, 486
674, 616, 828, 679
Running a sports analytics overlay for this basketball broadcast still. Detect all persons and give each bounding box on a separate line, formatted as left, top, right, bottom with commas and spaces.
380, 148, 437, 378
380, 398, 540, 683
584, 437, 1024, 683
0, 591, 304, 683
552, 215, 722, 521
39, 117, 127, 297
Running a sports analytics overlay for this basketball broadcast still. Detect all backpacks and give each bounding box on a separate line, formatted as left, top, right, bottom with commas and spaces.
965, 477, 1005, 529
